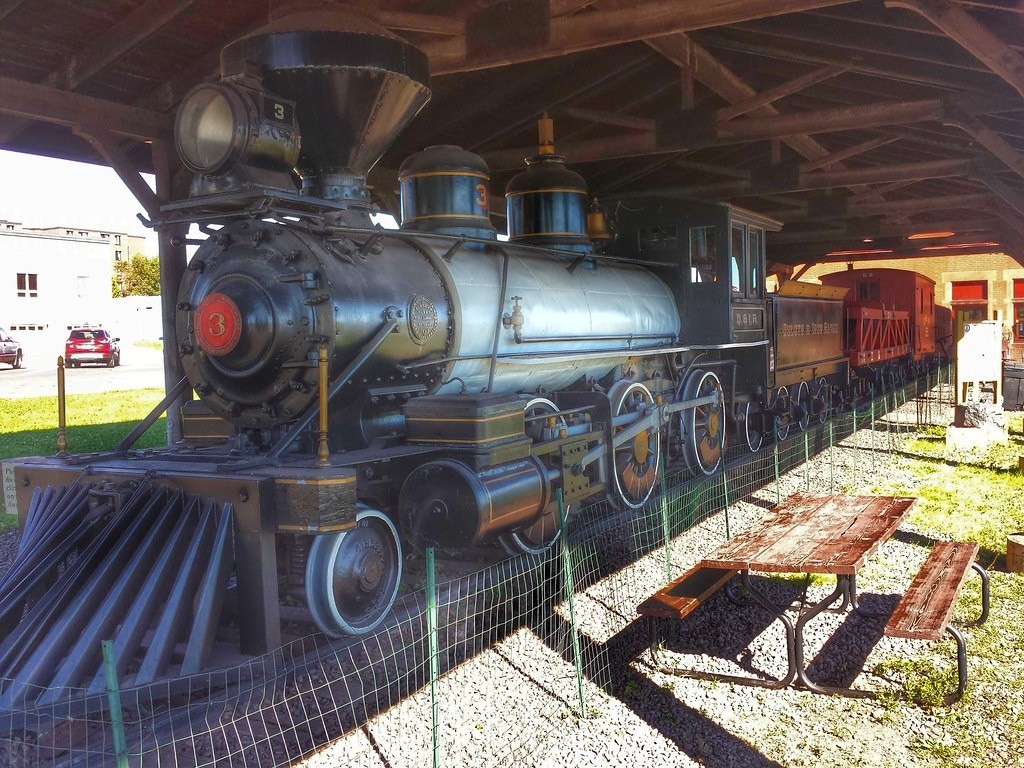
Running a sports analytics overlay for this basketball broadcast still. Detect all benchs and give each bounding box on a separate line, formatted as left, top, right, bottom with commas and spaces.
884, 541, 990, 703
638, 541, 739, 689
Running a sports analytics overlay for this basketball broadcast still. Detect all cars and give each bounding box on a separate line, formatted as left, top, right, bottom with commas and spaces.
65, 326, 120, 368
0, 326, 26, 369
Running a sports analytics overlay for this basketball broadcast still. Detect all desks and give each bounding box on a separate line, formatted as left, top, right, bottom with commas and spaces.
695, 490, 915, 701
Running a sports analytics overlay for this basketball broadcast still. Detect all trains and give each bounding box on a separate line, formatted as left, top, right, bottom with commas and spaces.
0, 28, 952, 641
